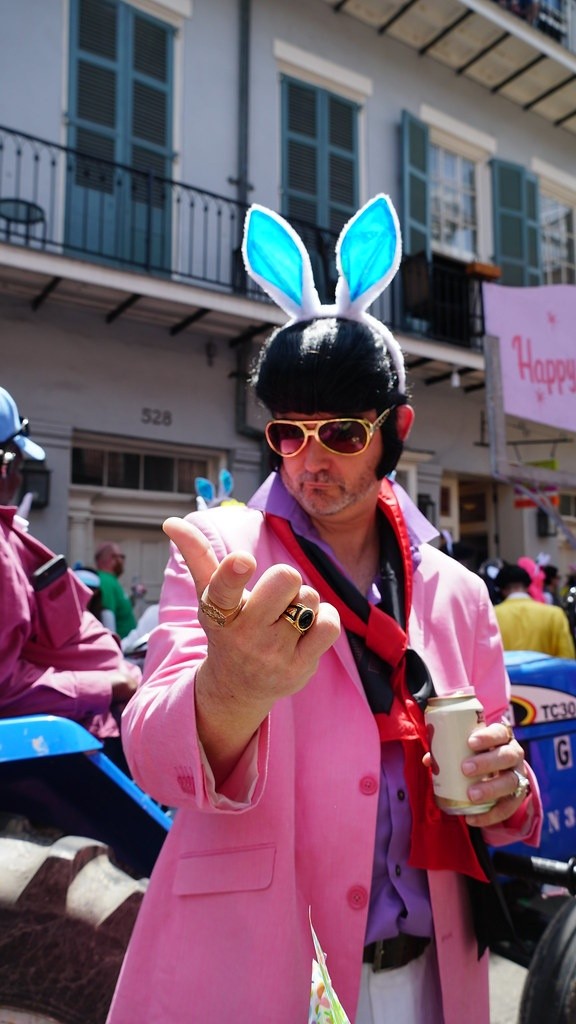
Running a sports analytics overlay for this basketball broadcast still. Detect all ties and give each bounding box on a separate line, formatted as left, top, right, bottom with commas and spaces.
264, 481, 487, 884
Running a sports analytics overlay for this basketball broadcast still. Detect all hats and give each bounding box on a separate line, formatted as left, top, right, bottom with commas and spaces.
74, 570, 100, 589
0, 386, 45, 461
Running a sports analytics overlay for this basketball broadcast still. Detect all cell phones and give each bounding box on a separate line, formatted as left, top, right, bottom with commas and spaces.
29, 553, 68, 591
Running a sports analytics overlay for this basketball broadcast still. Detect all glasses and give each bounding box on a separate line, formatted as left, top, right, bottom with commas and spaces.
266, 403, 397, 455
4, 415, 32, 444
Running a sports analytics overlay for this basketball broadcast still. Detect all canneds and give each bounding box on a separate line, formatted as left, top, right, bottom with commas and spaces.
423, 691, 498, 815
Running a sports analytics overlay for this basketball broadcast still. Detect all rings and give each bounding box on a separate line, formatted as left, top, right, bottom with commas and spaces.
511, 768, 530, 800
199, 584, 243, 627
282, 602, 315, 635
497, 722, 511, 743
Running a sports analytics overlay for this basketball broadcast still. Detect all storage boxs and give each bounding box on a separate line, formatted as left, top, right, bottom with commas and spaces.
491, 651, 576, 860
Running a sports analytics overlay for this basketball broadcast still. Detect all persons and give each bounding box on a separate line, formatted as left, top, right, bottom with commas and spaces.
0, 387, 144, 778
72, 542, 137, 639
104, 191, 546, 1023
440, 529, 575, 660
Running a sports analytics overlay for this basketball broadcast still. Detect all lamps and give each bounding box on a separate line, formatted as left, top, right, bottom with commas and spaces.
18, 456, 52, 513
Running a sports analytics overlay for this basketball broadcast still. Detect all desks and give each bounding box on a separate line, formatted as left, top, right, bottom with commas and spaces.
0, 199, 47, 251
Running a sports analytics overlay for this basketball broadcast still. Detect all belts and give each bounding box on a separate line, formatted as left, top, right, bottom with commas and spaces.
361, 933, 432, 973
